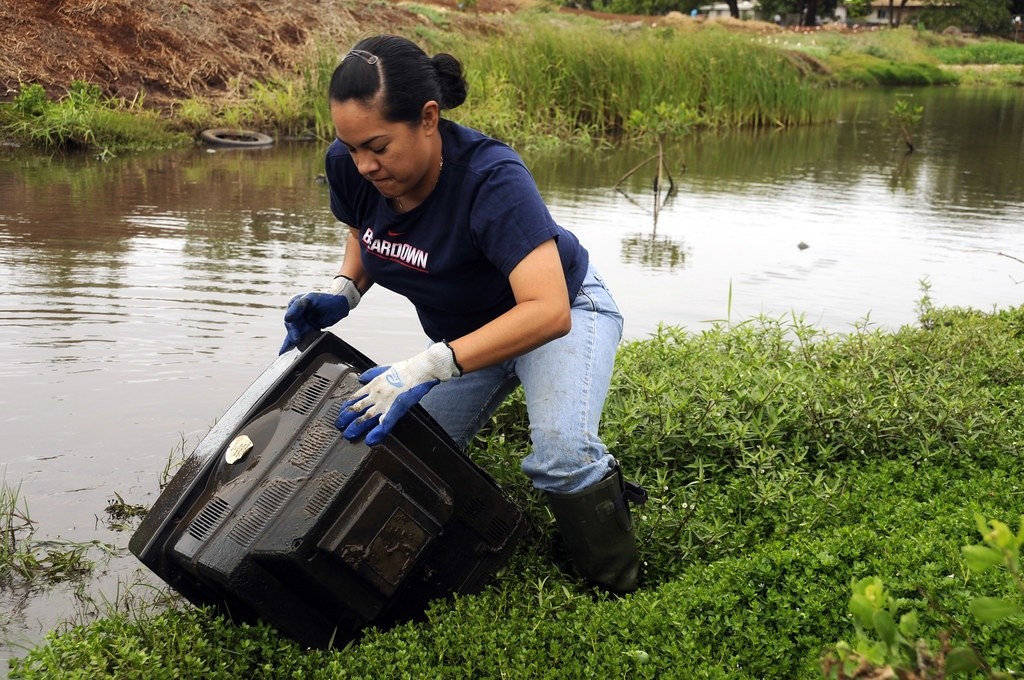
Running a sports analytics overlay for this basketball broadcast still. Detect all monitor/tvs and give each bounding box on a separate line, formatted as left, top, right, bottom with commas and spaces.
128, 330, 545, 652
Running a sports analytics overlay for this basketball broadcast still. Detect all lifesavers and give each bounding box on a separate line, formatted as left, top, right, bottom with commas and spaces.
200, 127, 275, 148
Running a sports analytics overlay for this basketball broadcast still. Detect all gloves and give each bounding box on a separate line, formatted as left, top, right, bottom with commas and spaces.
333, 339, 462, 447
278, 274, 364, 356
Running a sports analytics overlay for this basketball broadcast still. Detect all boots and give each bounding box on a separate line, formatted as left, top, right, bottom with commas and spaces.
543, 457, 650, 603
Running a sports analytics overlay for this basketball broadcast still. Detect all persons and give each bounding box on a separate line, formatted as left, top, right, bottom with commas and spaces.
278, 35, 646, 598
